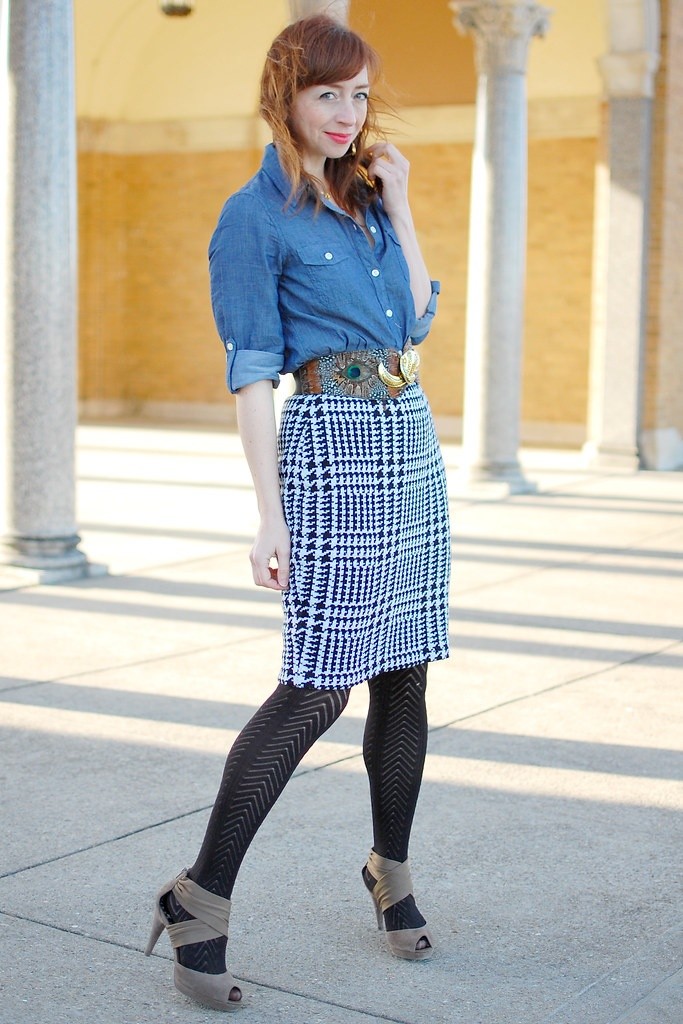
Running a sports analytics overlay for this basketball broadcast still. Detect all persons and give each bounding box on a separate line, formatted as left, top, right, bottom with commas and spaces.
144, 16, 451, 1011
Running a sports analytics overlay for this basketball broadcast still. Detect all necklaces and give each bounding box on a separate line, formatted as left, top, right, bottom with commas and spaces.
321, 192, 331, 199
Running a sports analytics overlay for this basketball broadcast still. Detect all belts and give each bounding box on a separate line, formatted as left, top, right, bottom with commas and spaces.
292, 335, 421, 399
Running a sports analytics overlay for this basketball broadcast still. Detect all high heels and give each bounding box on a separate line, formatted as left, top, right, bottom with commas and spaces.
144, 868, 244, 1013
362, 847, 434, 959
